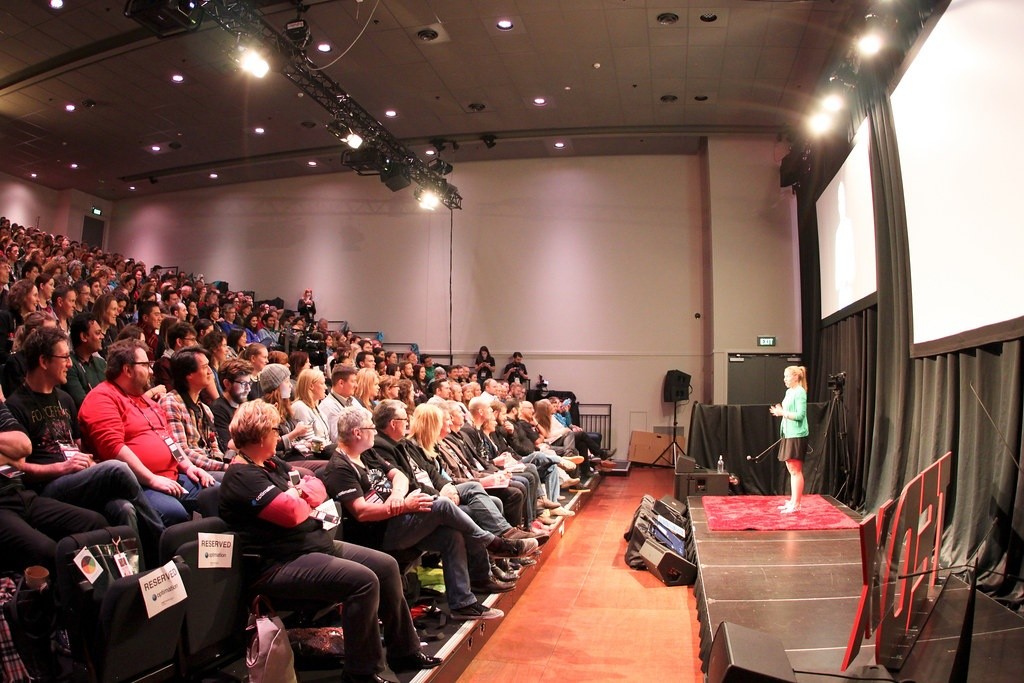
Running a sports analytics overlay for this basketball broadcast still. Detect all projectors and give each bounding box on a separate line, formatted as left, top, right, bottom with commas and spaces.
130, 0, 200, 33
344, 150, 391, 171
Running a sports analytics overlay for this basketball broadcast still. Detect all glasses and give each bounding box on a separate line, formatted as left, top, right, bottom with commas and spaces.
130, 360, 155, 368
352, 426, 376, 435
270, 428, 282, 437
389, 418, 408, 422
183, 337, 196, 341
45, 354, 71, 361
228, 379, 253, 388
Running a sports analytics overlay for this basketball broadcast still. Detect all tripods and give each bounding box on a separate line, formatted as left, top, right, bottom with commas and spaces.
809, 393, 853, 509
649, 402, 687, 469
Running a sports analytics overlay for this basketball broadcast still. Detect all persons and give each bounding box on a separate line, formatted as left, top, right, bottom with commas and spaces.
218, 397, 444, 683
371, 398, 550, 591
1, 215, 618, 575
324, 405, 539, 619
769, 365, 809, 514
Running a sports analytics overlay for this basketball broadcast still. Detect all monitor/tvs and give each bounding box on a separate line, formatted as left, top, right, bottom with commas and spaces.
653, 525, 685, 557
651, 499, 684, 531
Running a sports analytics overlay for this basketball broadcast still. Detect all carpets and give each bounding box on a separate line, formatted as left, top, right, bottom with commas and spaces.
701, 492, 861, 534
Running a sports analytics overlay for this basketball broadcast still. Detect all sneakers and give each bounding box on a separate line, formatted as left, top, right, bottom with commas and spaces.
450, 603, 505, 619
490, 538, 538, 559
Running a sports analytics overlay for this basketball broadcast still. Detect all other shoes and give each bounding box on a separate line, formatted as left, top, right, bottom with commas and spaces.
527, 447, 617, 535
781, 504, 801, 513
777, 500, 791, 509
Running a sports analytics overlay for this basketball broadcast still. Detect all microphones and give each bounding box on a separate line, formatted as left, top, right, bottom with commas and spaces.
746, 456, 758, 464
309, 332, 323, 340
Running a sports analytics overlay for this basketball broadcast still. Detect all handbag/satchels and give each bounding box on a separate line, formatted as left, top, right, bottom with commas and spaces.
244, 594, 297, 683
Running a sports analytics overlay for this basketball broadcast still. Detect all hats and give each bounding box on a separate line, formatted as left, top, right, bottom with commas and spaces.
259, 364, 291, 392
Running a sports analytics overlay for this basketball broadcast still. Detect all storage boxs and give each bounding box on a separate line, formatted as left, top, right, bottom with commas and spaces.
626, 430, 686, 468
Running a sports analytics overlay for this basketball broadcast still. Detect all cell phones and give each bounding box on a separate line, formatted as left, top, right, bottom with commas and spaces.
288, 471, 300, 486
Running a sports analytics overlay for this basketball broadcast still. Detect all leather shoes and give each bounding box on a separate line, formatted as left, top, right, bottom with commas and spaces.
491, 564, 520, 580
394, 649, 441, 668
341, 669, 398, 683
471, 576, 516, 592
502, 529, 549, 547
510, 557, 535, 564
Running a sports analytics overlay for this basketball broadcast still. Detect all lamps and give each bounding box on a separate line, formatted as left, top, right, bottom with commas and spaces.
325, 112, 364, 150
413, 186, 440, 211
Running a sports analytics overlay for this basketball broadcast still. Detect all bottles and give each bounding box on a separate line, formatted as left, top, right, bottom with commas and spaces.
560, 398, 572, 410
717, 454, 724, 473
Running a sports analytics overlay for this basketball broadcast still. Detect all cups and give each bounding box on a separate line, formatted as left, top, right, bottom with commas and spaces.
25, 565, 50, 589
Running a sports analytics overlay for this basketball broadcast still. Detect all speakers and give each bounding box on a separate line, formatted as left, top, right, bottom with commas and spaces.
709, 621, 797, 683
675, 470, 729, 502
665, 369, 692, 403
381, 162, 411, 192
675, 454, 696, 472
659, 495, 687, 515
638, 539, 699, 587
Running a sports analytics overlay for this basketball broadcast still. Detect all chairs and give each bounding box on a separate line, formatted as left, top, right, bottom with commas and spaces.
1, 457, 342, 683
